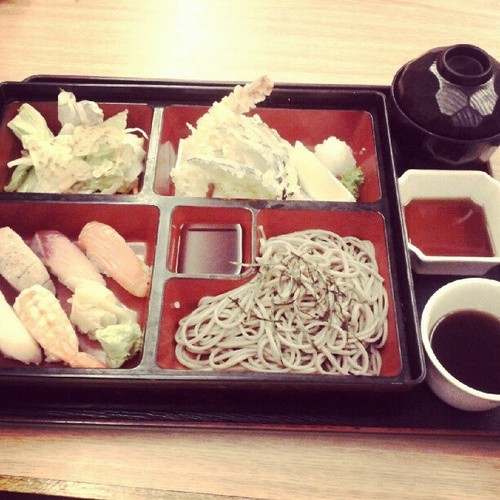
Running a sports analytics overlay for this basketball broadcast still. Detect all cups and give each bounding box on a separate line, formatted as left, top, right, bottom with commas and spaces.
392, 62, 500, 171
420, 277, 500, 411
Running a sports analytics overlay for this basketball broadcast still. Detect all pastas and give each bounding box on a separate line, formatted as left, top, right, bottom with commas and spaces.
173, 227, 388, 377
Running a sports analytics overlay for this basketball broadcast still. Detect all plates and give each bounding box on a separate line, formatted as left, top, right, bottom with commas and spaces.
0, 81, 427, 394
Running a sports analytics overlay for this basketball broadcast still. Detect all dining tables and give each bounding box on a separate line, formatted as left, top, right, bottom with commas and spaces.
0, 1, 500, 500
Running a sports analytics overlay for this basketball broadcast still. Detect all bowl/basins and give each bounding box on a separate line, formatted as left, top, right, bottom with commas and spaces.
398, 44, 500, 139
397, 170, 500, 277
